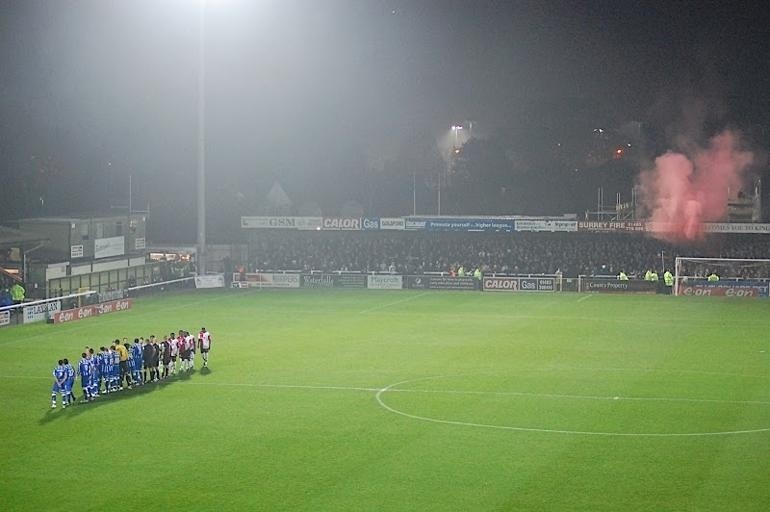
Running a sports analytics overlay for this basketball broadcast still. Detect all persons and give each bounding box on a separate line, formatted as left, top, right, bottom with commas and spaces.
51, 327, 212, 409
0, 246, 195, 310
220, 230, 769, 295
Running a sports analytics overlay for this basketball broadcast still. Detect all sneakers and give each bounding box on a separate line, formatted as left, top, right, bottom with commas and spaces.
127, 374, 159, 390
162, 364, 207, 377
51, 398, 76, 409
84, 388, 122, 404
